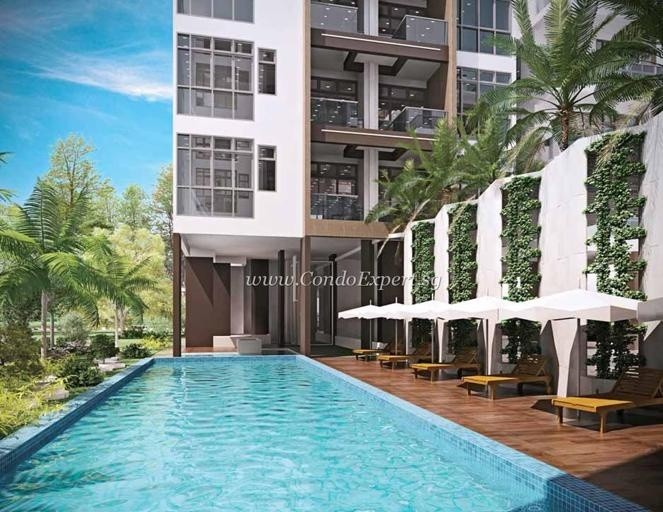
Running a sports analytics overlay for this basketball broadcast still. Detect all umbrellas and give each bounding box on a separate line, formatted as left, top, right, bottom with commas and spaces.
499, 278, 645, 422
360, 297, 410, 354
397, 294, 470, 363
337, 299, 382, 349
439, 288, 515, 392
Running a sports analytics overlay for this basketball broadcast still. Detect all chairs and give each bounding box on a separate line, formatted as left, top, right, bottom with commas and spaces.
352, 338, 482, 383
460, 353, 553, 402
549, 365, 663, 434
